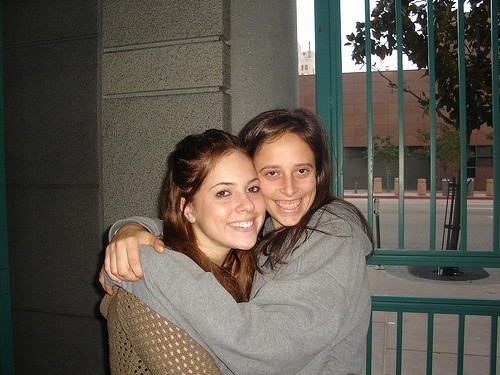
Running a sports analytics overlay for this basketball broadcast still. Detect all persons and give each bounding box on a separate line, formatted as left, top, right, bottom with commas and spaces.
97, 129, 266, 375
100, 107, 373, 375
